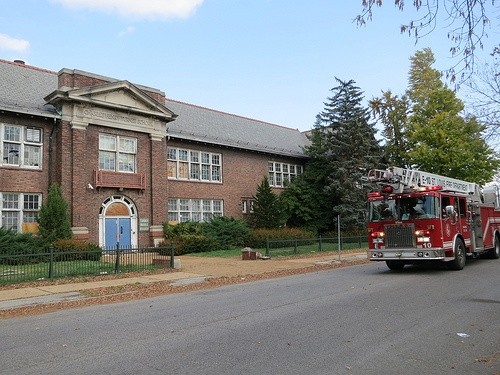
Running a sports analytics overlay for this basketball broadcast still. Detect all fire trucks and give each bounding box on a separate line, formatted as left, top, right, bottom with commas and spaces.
359, 165, 500, 269
